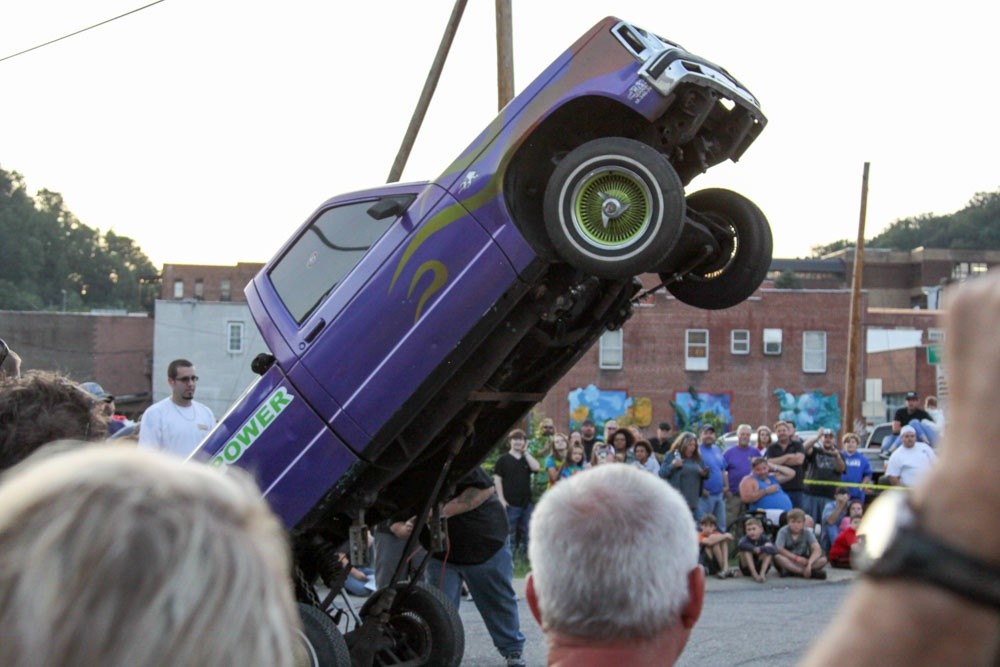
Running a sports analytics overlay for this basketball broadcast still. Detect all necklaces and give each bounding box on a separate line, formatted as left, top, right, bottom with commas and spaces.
167, 398, 198, 423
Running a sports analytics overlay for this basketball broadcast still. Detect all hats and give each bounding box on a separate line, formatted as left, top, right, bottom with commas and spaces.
905, 391, 918, 400
823, 429, 833, 436
79, 382, 110, 402
900, 425, 916, 436
596, 444, 615, 455
703, 423, 715, 433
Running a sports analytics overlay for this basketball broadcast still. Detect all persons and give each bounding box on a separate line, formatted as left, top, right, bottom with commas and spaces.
793, 264, 999, 666
524, 462, 706, 667
326, 553, 470, 597
0, 436, 310, 666
362, 465, 526, 666
879, 391, 946, 489
480, 413, 873, 582
0, 358, 221, 471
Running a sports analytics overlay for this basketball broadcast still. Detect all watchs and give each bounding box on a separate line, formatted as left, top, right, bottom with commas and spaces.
853, 483, 1000, 612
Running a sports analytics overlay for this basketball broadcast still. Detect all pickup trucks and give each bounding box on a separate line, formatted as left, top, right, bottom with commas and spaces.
855, 420, 895, 484
182, 12, 774, 667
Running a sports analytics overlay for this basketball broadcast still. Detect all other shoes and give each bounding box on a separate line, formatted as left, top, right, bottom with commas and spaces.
505, 652, 526, 667
879, 451, 891, 460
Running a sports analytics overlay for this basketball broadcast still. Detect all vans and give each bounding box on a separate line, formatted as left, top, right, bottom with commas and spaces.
713, 429, 821, 456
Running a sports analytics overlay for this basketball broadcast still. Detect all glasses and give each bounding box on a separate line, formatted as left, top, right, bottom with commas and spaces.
106, 396, 114, 404
175, 375, 199, 384
